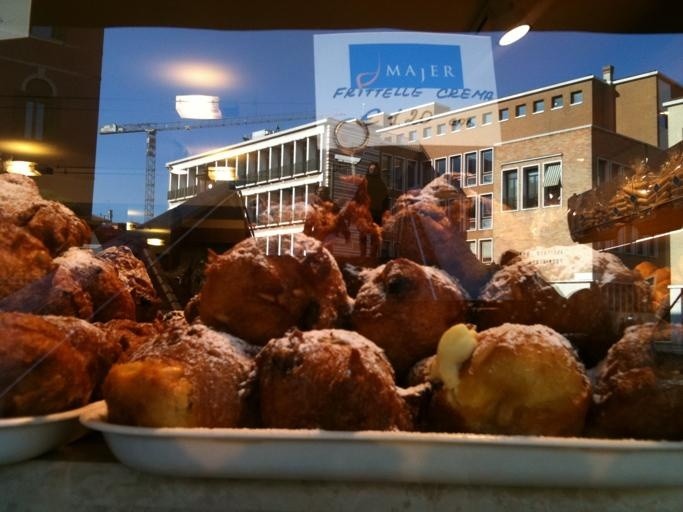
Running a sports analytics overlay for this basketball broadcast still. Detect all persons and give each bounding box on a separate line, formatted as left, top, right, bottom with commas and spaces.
303, 161, 389, 257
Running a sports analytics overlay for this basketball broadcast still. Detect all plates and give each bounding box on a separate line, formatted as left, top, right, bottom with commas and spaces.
77, 400, 683, 490
1, 400, 102, 464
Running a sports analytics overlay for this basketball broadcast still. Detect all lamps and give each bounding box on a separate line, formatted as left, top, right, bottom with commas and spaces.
499, 22, 530, 46
194, 166, 240, 185
1, 158, 52, 176
